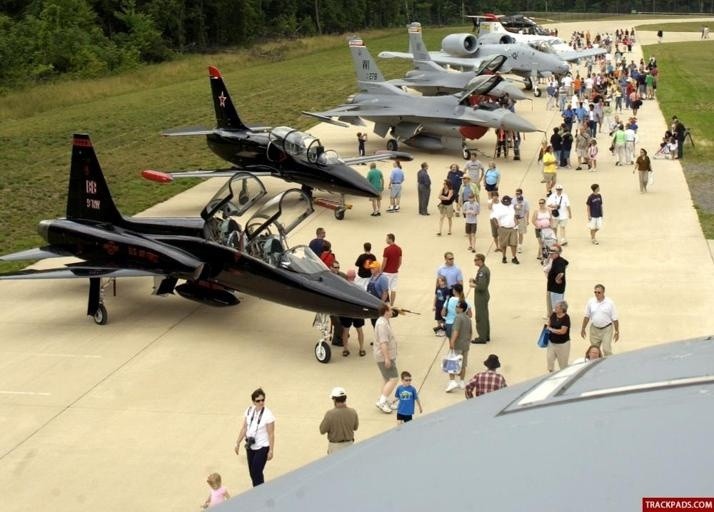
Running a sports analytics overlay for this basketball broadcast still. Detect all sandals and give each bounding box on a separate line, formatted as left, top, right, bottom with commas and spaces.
360, 351, 366, 356
343, 350, 350, 356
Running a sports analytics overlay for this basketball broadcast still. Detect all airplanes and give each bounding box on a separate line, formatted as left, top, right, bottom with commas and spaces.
0, 134, 417, 362
142, 67, 412, 218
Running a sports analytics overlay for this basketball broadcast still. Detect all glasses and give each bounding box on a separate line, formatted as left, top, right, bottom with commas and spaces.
255, 398, 265, 402
594, 289, 602, 294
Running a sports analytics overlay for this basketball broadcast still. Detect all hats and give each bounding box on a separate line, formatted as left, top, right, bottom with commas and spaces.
331, 388, 347, 399
347, 270, 356, 278
460, 174, 471, 179
556, 184, 563, 190
484, 355, 501, 369
369, 261, 382, 269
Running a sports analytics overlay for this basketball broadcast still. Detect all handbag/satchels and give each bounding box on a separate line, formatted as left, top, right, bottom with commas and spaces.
437, 202, 444, 215
491, 218, 499, 231
551, 209, 560, 218
539, 325, 548, 347
441, 354, 464, 374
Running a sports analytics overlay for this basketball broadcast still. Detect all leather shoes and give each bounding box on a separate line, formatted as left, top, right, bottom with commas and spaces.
471, 337, 489, 343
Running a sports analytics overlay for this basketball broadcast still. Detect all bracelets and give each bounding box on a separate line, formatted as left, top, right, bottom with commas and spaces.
234, 441, 241, 450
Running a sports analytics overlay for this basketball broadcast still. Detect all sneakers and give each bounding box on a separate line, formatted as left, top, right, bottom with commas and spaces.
592, 239, 598, 244
502, 247, 522, 263
370, 209, 400, 216
467, 246, 475, 252
561, 241, 568, 245
446, 379, 468, 392
376, 400, 397, 413
437, 232, 451, 236
576, 162, 597, 172
617, 159, 635, 167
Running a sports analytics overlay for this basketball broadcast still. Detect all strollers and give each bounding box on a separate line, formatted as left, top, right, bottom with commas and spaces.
539, 225, 560, 265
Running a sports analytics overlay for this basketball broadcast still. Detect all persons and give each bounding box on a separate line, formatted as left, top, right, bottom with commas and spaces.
318, 385, 360, 458
305, 19, 713, 366
439, 300, 474, 393
537, 299, 570, 374
234, 387, 277, 489
372, 302, 400, 415
200, 471, 232, 509
464, 354, 510, 401
391, 369, 426, 428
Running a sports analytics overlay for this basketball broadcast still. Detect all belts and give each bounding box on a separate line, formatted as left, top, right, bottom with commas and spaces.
500, 226, 515, 231
329, 439, 354, 443
391, 180, 400, 185
594, 323, 612, 329
